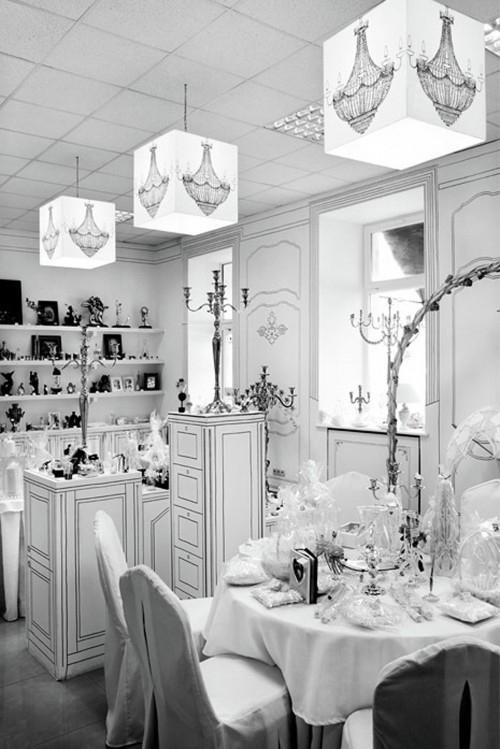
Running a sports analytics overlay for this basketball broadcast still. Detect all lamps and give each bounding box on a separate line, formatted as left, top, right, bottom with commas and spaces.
133, 84, 239, 238
322, 3, 487, 174
39, 154, 118, 271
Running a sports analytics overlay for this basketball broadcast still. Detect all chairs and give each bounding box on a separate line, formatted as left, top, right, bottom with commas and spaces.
88, 505, 215, 747
104, 565, 288, 748
372, 638, 500, 749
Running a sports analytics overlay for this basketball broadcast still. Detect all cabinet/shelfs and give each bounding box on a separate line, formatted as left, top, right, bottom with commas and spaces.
169, 411, 267, 601
24, 468, 146, 680
